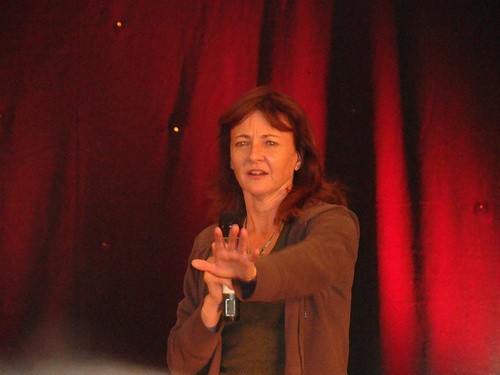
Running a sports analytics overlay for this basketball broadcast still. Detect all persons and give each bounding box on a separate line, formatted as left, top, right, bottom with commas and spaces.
166, 87, 360, 375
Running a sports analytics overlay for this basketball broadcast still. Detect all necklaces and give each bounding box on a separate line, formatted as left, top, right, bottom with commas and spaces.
242, 216, 284, 257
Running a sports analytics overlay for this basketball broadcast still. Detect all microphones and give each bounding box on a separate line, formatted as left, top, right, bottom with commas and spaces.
218, 211, 244, 321
